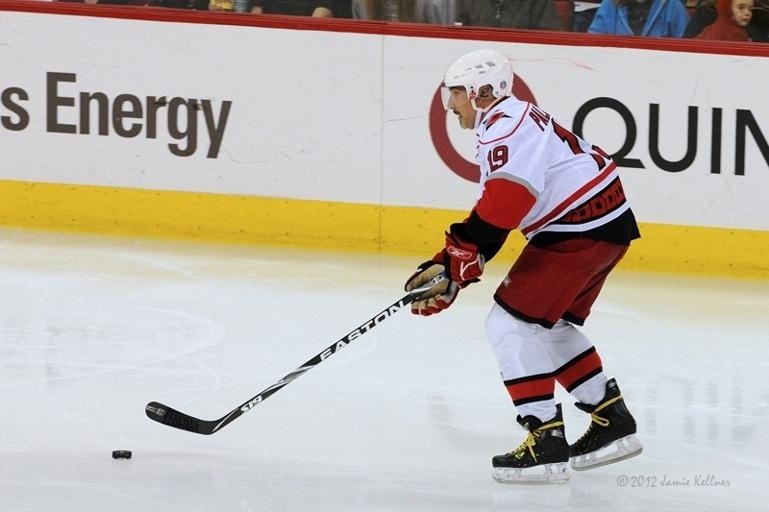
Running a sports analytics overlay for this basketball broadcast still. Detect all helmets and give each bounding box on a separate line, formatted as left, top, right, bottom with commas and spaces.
442, 50, 513, 113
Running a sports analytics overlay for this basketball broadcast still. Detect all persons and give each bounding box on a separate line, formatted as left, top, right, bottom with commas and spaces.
403, 49, 636, 467
61, 0, 767, 46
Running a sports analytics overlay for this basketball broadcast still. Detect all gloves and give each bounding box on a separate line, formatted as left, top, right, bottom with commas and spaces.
446, 217, 486, 280
404, 246, 460, 316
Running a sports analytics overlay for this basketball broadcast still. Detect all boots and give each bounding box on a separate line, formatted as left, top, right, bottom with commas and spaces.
569, 378, 635, 457
492, 403, 569, 468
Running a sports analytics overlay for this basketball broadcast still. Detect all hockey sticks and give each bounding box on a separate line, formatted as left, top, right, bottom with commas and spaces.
146, 271, 449, 435
